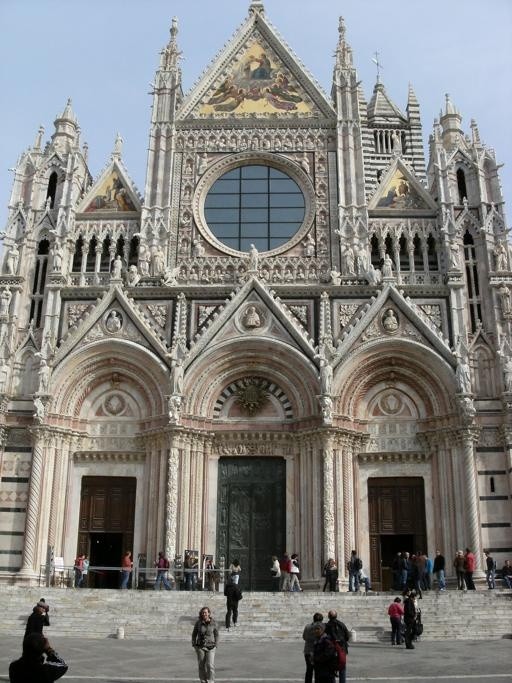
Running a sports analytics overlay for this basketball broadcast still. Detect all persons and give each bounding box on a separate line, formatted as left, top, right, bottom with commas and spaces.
384, 308, 399, 329
271, 552, 304, 592
318, 357, 333, 395
53, 242, 65, 270
105, 310, 122, 332
246, 305, 261, 328
192, 234, 207, 259
192, 605, 219, 683
294, 152, 310, 173
122, 552, 133, 589
7, 243, 19, 277
323, 551, 372, 593
503, 355, 512, 391
168, 358, 184, 394
110, 245, 168, 288
9, 599, 68, 681
388, 549, 512, 599
391, 130, 402, 149
115, 133, 124, 151
388, 590, 421, 649
448, 240, 463, 270
223, 579, 243, 628
493, 239, 507, 273
154, 553, 241, 592
76, 553, 90, 588
330, 241, 394, 287
209, 53, 302, 112
455, 356, 472, 392
300, 233, 316, 259
302, 611, 351, 682
247, 243, 260, 271
497, 280, 511, 315
37, 359, 51, 393
196, 152, 215, 175
0, 284, 13, 316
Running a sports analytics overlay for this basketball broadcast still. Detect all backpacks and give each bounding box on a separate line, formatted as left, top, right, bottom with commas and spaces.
320, 637, 346, 671
354, 558, 362, 570
162, 557, 169, 571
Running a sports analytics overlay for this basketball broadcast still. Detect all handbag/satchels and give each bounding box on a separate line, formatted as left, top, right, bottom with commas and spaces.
412, 622, 423, 636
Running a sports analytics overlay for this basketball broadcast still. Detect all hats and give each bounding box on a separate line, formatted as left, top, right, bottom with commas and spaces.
37, 598, 47, 609
